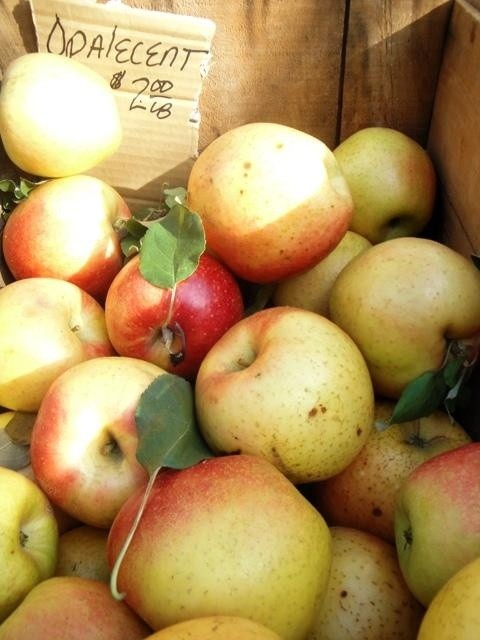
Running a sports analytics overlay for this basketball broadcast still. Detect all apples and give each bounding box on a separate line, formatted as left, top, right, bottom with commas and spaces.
1, 51, 480, 640
